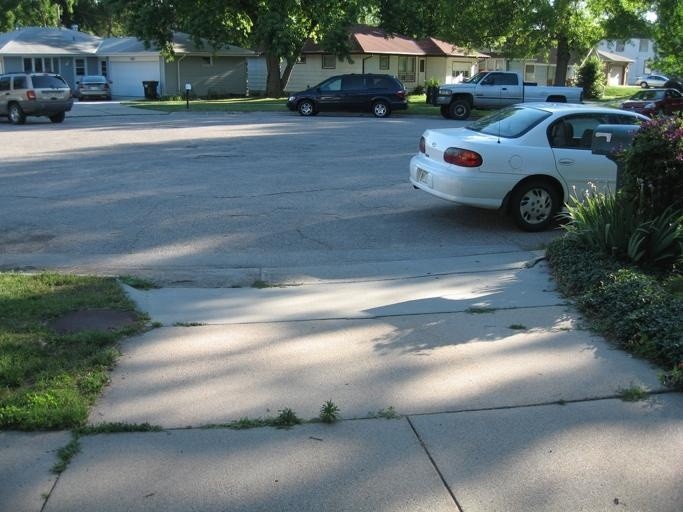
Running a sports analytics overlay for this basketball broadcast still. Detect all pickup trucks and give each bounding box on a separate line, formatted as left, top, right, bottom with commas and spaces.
422, 70, 586, 121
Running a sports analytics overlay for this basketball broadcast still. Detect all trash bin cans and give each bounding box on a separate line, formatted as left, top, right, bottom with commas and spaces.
142, 80, 159, 98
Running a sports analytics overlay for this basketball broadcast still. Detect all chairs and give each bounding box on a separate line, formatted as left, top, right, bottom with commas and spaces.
562, 122, 593, 149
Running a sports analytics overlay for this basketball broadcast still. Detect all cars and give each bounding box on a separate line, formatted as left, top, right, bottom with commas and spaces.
634, 75, 668, 88
408, 103, 653, 231
620, 88, 682, 120
75, 76, 113, 101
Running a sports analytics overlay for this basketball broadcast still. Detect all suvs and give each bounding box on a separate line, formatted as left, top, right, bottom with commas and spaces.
0, 71, 73, 124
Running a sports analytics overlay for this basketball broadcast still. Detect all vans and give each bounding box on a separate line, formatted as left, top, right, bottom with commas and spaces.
285, 73, 409, 117
